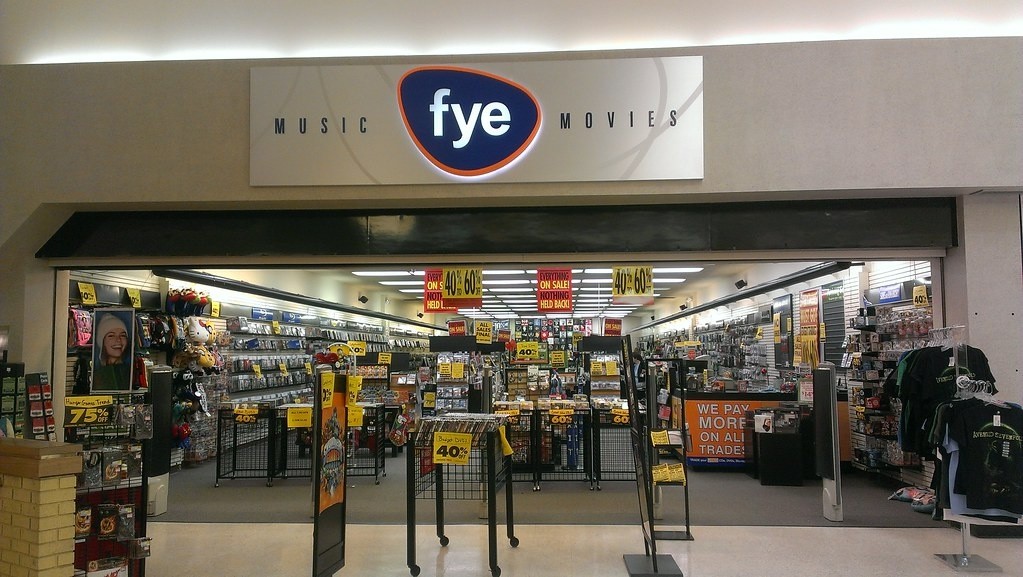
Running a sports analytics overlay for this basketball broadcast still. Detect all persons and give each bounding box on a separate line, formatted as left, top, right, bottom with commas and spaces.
92, 313, 131, 392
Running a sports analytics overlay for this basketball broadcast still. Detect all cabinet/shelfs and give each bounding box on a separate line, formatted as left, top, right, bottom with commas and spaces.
851, 299, 934, 471
347, 335, 636, 473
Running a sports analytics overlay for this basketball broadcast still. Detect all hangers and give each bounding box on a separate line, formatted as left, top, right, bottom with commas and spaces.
923, 325, 1011, 408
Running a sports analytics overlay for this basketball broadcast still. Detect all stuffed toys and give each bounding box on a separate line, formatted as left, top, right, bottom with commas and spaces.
172, 317, 221, 441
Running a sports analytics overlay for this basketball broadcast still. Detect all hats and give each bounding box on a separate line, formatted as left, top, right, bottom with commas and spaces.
96, 313, 127, 366
